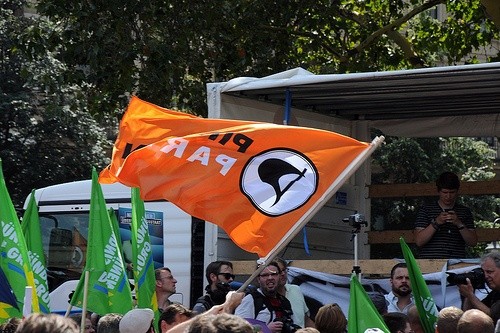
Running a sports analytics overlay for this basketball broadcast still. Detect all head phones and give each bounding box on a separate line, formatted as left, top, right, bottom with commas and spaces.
205, 285, 224, 304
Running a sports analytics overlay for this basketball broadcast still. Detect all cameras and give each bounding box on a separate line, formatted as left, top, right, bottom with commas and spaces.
446, 268, 486, 289
342, 210, 368, 228
273, 316, 302, 333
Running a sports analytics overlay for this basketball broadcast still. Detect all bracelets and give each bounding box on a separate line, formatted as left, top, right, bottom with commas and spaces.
458, 224, 466, 231
431, 218, 440, 230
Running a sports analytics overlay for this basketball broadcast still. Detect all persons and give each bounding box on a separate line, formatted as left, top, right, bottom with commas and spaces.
193, 261, 235, 315
273, 256, 315, 329
0, 292, 500, 333
155, 267, 182, 311
460, 252, 500, 323
235, 261, 293, 333
413, 172, 478, 259
383, 263, 415, 315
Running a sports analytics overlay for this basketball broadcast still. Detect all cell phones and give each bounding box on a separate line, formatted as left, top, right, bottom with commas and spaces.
443, 209, 452, 215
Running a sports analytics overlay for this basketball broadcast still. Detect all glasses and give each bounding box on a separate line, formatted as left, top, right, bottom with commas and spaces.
216, 273, 235, 280
281, 268, 290, 273
79, 326, 96, 331
156, 276, 173, 280
259, 272, 279, 277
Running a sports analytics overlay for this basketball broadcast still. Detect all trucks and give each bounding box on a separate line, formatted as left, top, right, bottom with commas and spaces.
21, 60, 499, 319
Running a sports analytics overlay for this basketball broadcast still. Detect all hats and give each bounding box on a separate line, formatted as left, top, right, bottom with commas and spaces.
119, 308, 154, 333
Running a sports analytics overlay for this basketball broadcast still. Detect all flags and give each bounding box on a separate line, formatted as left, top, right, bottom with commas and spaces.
399, 237, 439, 333
98, 95, 372, 265
131, 188, 161, 333
69, 167, 134, 316
347, 272, 391, 333
0, 161, 51, 325
